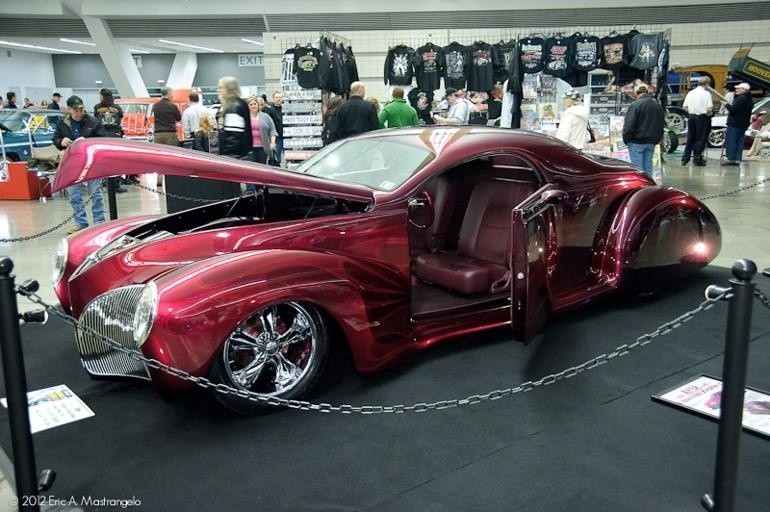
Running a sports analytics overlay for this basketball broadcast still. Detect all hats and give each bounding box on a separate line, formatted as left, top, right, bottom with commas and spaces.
734, 83, 750, 90
68, 96, 85, 108
635, 84, 649, 94
442, 88, 456, 99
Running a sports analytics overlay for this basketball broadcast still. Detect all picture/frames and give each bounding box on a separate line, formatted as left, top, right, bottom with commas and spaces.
650, 372, 769, 441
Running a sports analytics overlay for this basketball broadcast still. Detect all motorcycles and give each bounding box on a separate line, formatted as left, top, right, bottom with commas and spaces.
662, 126, 678, 154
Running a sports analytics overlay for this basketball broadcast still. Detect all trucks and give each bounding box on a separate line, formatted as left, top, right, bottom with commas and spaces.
664, 41, 770, 138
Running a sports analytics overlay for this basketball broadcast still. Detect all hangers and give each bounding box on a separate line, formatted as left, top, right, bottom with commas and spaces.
321, 29, 352, 44
293, 39, 313, 49
383, 21, 664, 48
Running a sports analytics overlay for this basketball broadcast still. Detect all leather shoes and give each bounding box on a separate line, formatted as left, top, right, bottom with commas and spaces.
682, 161, 740, 166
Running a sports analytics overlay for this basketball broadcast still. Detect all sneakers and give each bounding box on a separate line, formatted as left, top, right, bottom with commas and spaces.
67, 224, 80, 233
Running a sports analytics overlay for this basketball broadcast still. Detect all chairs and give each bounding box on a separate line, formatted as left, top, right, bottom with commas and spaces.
413, 177, 537, 297
409, 171, 460, 257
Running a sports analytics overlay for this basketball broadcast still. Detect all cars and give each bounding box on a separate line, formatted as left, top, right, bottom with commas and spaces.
0, 106, 70, 169
707, 85, 770, 150
114, 88, 191, 142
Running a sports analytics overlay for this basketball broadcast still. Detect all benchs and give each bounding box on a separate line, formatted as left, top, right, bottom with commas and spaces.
0, 160, 51, 202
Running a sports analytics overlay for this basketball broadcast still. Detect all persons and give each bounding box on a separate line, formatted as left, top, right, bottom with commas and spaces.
52, 95, 107, 234
378, 88, 418, 128
680, 76, 714, 167
0, 87, 283, 195
721, 85, 733, 108
720, 83, 753, 166
215, 76, 252, 161
622, 85, 663, 178
556, 91, 589, 151
323, 97, 344, 147
435, 88, 472, 124
364, 96, 380, 111
334, 82, 378, 143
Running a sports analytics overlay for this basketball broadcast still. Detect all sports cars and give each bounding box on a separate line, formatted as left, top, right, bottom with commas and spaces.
50, 124, 721, 417
